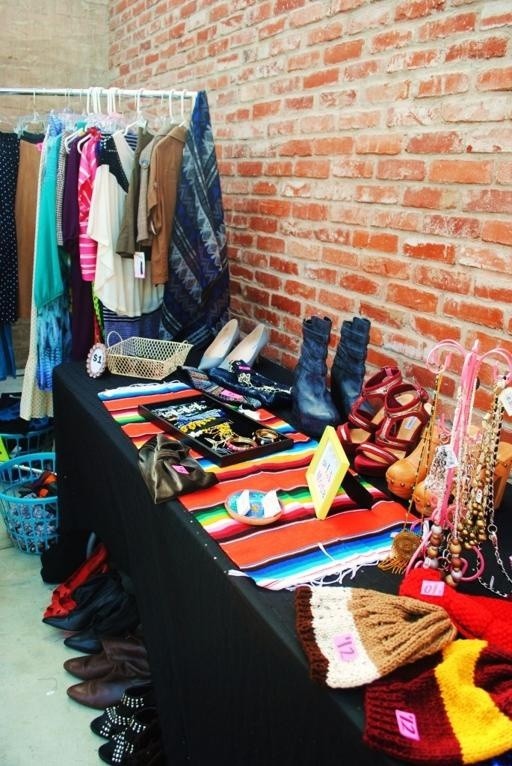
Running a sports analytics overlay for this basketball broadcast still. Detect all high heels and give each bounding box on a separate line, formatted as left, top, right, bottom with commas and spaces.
40, 528, 140, 653
385, 418, 512, 521
198, 318, 269, 373
90, 687, 165, 765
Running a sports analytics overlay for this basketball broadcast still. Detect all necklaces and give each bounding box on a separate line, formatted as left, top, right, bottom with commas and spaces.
378, 358, 512, 600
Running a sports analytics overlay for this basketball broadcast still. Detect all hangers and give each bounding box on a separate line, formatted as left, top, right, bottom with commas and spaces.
5, 84, 193, 156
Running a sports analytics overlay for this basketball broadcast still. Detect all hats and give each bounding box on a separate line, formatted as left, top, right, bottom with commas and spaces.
295, 585, 458, 691
399, 568, 512, 654
362, 638, 512, 766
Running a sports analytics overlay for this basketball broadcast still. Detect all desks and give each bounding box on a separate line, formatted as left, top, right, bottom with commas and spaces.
50, 331, 512, 766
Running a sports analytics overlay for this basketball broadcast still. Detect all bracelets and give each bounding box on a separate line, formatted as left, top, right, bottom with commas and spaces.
154, 399, 281, 458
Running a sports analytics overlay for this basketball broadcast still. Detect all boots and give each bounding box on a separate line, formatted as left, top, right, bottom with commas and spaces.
62, 635, 153, 709
290, 316, 378, 424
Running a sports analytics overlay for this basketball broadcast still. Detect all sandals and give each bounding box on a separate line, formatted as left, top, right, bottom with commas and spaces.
335, 365, 430, 478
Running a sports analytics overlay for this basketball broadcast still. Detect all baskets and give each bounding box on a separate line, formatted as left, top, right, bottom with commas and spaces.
1, 425, 56, 464
104, 330, 195, 381
0, 451, 68, 557
